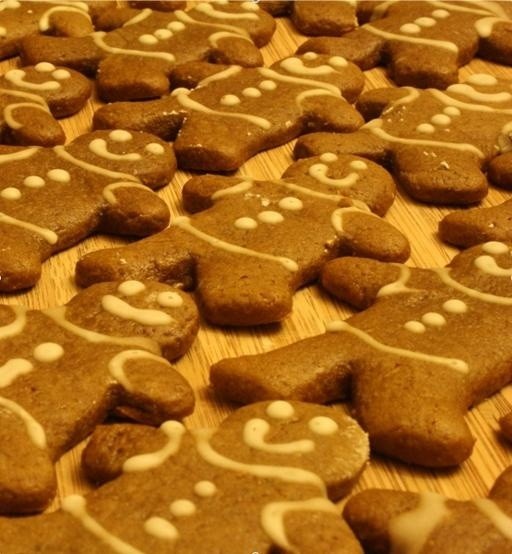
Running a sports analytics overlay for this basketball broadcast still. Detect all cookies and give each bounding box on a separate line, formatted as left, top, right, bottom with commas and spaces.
342, 462, 512, 554
92, 50, 363, 173
0, 128, 177, 296
293, 70, 512, 205
438, 195, 512, 249
207, 239, 512, 471
0, 1, 116, 61
0, 62, 93, 147
295, 1, 512, 89
1, 280, 200, 517
19, 1, 276, 103
73, 150, 411, 330
2, 399, 372, 552
257, 2, 384, 37
